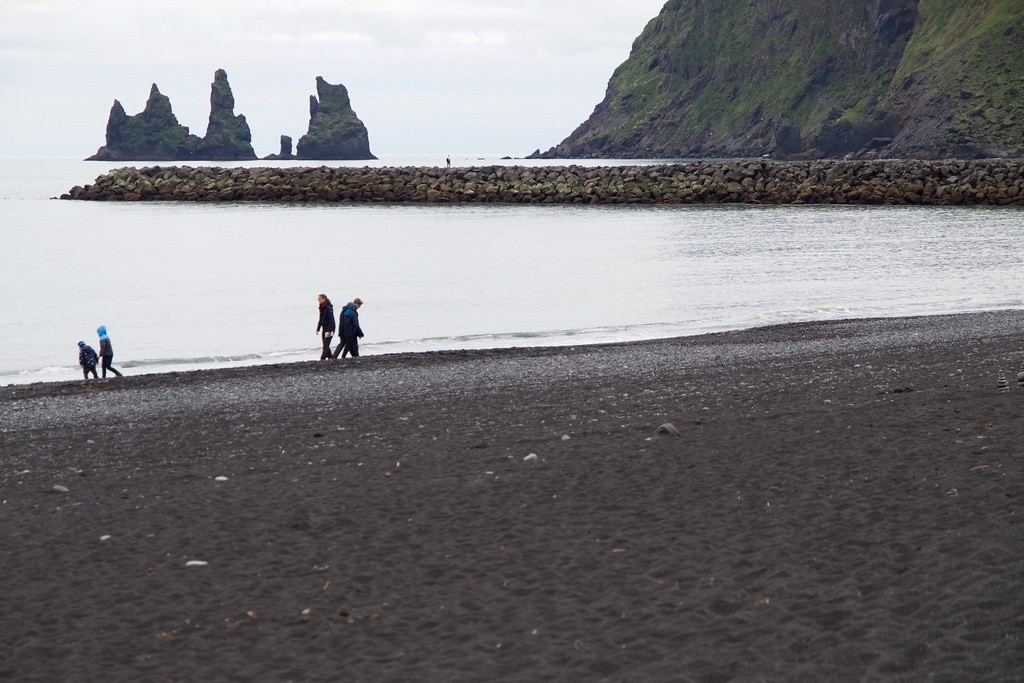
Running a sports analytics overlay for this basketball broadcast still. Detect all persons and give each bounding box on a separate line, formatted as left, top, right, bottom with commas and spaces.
97, 326, 122, 378
316, 294, 335, 361
78, 341, 99, 380
333, 299, 364, 358
446, 155, 451, 167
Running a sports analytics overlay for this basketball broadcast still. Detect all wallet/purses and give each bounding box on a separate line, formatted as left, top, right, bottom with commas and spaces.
325, 332, 331, 338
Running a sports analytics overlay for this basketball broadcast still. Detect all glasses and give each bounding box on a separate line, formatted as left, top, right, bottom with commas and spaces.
319, 298, 321, 300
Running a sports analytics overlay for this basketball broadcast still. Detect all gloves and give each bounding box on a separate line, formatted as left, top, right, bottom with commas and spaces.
316, 329, 320, 335
